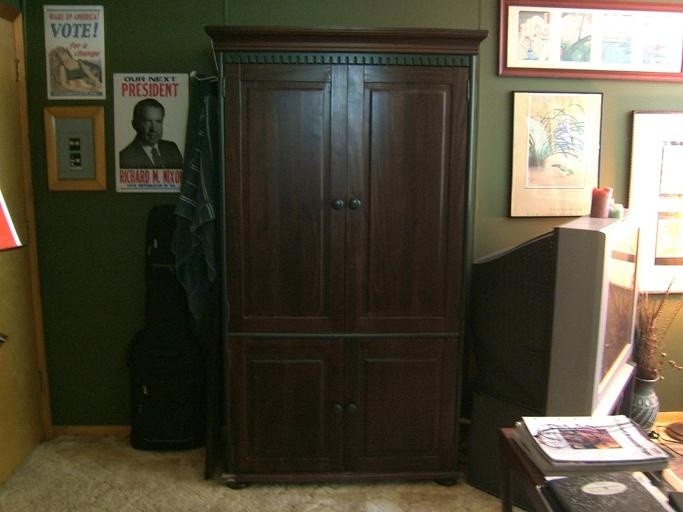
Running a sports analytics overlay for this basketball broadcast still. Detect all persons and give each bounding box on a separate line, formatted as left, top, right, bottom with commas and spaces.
49, 47, 102, 92
120, 99, 184, 169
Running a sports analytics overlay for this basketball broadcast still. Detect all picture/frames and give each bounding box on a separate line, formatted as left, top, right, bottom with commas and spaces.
496, 0, 682, 85
628, 109, 683, 295
509, 90, 604, 218
42, 105, 107, 191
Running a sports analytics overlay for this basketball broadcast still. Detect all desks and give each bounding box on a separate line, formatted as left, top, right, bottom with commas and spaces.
499, 410, 683, 512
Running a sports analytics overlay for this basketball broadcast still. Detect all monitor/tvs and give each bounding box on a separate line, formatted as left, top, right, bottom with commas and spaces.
461, 208, 649, 416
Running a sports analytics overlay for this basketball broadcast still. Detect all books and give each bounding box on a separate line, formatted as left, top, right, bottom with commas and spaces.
514, 413, 683, 512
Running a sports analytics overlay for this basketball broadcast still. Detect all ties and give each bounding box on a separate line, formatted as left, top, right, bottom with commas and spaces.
151, 147, 162, 168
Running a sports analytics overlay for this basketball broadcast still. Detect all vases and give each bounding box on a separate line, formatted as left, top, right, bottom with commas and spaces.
626, 378, 661, 430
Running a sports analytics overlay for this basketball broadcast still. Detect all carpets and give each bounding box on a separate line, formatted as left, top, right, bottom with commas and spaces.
1, 438, 518, 511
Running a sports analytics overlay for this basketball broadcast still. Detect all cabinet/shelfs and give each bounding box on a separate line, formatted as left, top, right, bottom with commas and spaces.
210, 24, 490, 489
